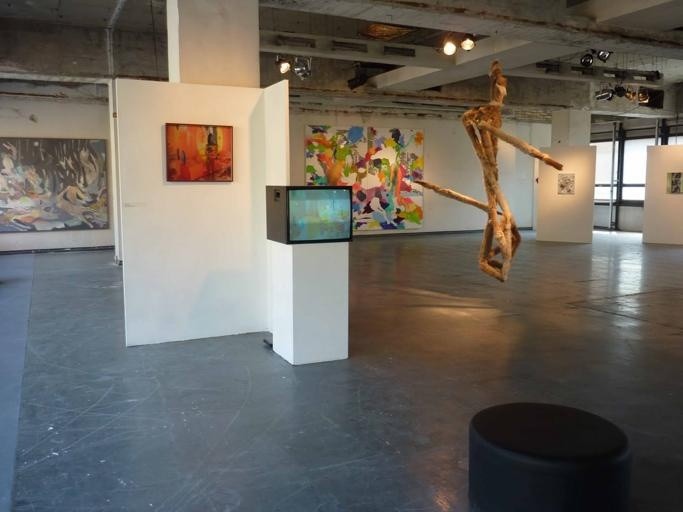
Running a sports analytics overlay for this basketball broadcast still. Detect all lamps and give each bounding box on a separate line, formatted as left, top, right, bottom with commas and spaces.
441, 32, 475, 56
579, 49, 611, 67
595, 83, 651, 105
275, 53, 312, 80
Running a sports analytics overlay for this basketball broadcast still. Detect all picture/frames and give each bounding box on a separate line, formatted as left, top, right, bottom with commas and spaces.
0, 136, 109, 233
164, 122, 234, 182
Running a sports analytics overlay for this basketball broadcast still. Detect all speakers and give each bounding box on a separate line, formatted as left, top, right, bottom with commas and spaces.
639, 86, 664, 108
